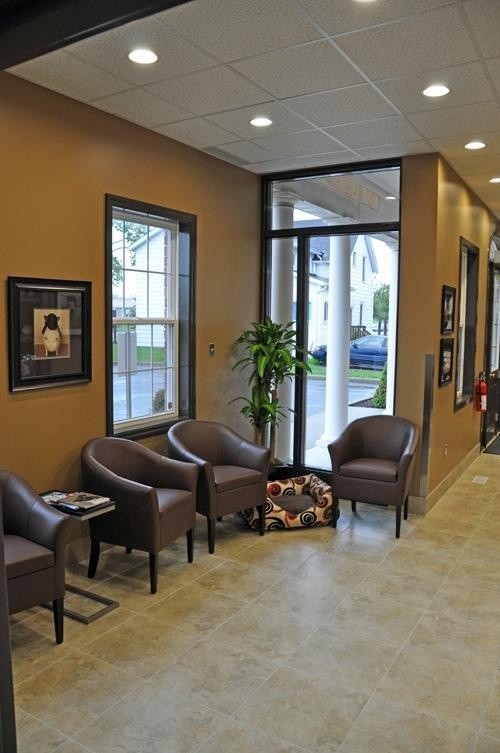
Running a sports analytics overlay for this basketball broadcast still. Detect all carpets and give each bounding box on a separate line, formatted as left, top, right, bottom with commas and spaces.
483, 432, 500, 456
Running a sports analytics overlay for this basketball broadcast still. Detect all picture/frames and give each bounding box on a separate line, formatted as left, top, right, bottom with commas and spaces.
8, 276, 91, 392
439, 338, 453, 386
440, 285, 456, 334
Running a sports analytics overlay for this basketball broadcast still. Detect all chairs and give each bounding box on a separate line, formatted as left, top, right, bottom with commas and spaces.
167, 419, 271, 554
81, 436, 199, 593
327, 415, 420, 539
0, 468, 71, 643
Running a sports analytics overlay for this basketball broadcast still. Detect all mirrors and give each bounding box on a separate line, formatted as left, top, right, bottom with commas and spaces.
454, 235, 480, 413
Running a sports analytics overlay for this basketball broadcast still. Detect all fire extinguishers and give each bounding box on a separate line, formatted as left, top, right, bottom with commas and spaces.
475, 372, 487, 412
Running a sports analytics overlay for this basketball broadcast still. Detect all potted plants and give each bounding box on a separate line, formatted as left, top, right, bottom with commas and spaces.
230, 315, 318, 481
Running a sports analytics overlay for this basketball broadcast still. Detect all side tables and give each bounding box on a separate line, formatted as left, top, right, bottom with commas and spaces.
39, 490, 120, 624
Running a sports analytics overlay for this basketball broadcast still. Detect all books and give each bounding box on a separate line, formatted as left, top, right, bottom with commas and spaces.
37, 487, 116, 521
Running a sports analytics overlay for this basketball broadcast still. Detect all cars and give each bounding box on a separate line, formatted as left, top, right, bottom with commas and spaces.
313, 334, 388, 367
